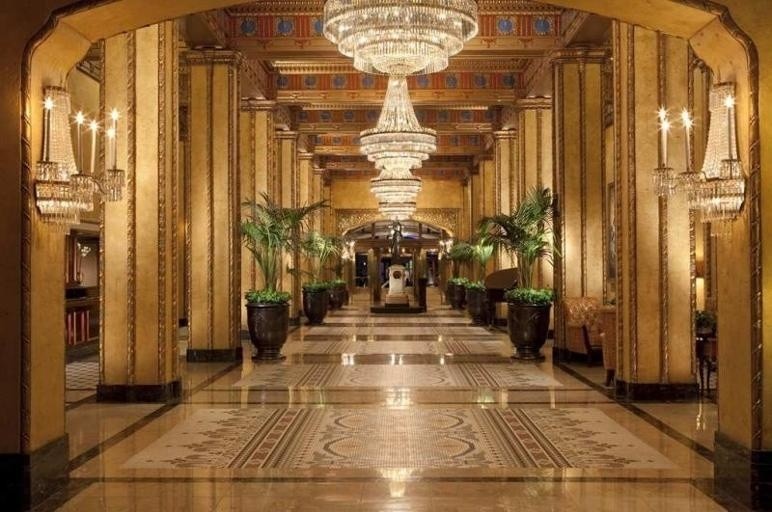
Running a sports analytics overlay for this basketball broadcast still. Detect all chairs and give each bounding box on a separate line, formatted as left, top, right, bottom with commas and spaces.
564, 297, 618, 387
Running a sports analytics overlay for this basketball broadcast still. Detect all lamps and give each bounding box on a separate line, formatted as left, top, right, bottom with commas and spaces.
322, 0, 478, 218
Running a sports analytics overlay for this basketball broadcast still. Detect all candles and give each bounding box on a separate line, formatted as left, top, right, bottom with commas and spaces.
659, 94, 734, 172
43, 95, 121, 174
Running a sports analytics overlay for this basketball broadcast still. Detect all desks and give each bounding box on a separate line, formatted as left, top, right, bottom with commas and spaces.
696, 328, 717, 397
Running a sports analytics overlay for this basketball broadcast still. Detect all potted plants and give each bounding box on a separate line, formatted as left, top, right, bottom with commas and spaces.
442, 183, 564, 361
696, 309, 714, 337
234, 190, 348, 361
